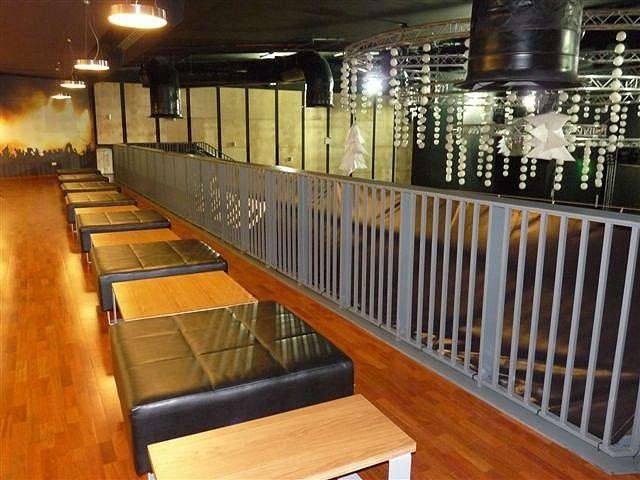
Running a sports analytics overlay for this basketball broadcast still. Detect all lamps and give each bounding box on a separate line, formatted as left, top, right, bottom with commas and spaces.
50, 55, 72, 100
72, 0, 110, 71
59, 32, 87, 89
105, 0, 167, 30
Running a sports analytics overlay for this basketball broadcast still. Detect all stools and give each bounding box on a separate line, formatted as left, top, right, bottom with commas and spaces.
105, 269, 260, 321
75, 208, 172, 238
88, 236, 232, 311
85, 226, 184, 252
59, 168, 143, 211
105, 297, 350, 470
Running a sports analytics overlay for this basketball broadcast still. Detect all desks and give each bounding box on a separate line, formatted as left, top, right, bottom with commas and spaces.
148, 393, 418, 479
